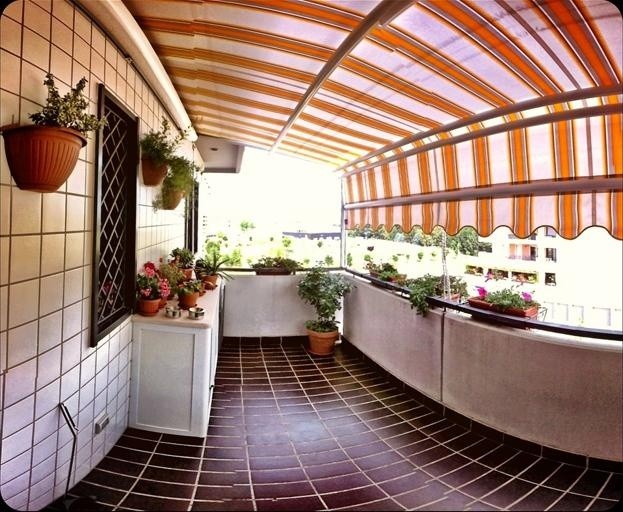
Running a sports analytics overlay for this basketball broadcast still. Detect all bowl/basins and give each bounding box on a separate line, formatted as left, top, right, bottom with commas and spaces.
166, 307, 204, 320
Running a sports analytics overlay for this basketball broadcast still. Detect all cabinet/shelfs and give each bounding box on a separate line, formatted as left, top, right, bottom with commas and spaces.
128, 285, 220, 438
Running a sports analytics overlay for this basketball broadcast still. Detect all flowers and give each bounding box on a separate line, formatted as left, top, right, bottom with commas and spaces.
137, 262, 172, 299
476, 273, 541, 310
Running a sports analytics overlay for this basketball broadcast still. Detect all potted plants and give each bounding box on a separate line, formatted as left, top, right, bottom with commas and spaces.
159, 248, 235, 309
296, 261, 357, 357
0, 74, 110, 193
363, 255, 407, 290
252, 257, 303, 275
398, 273, 469, 318
152, 156, 204, 213
139, 116, 203, 185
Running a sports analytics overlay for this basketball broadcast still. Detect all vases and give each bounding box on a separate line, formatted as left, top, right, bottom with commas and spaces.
136, 299, 161, 317
468, 295, 538, 330
